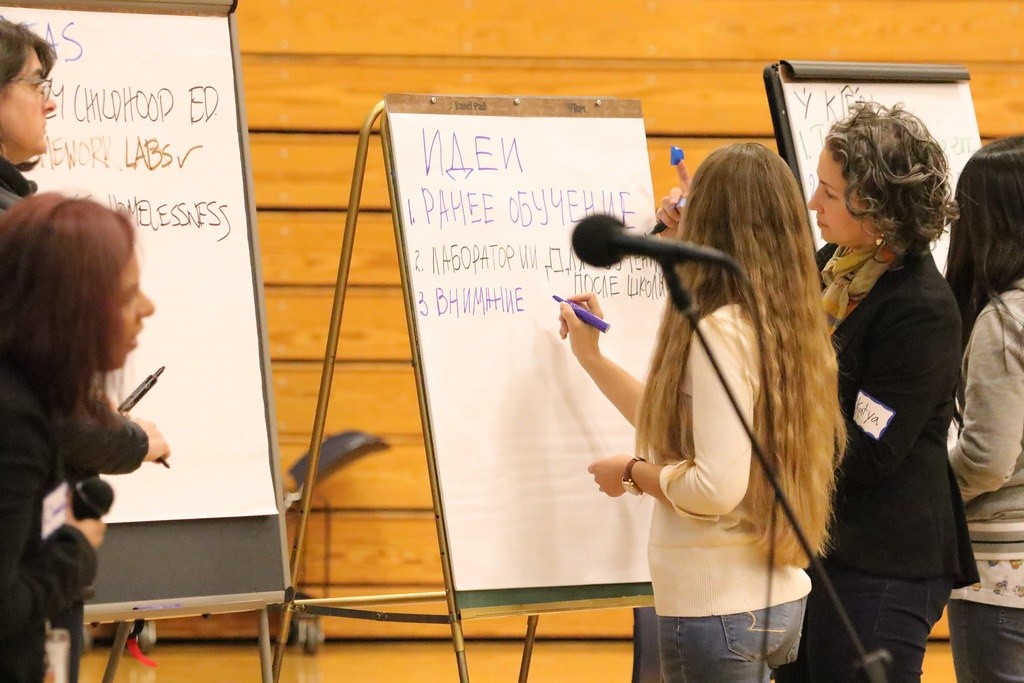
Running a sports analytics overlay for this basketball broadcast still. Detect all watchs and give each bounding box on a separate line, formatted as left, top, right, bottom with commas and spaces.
621, 457, 646, 496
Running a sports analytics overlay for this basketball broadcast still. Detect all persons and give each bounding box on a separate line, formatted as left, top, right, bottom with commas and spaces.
560, 142, 846, 683
946, 135, 1024, 683
661, 101, 978, 683
0, 19, 170, 683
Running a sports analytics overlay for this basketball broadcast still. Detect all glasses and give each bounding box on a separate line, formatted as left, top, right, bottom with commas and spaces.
0, 74, 52, 102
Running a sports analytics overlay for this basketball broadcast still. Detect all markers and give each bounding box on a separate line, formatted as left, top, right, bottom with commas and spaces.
120, 411, 170, 470
552, 295, 611, 335
647, 222, 668, 237
116, 365, 166, 415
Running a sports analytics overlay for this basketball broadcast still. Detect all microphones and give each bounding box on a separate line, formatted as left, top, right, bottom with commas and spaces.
571, 214, 737, 269
72, 479, 116, 522
